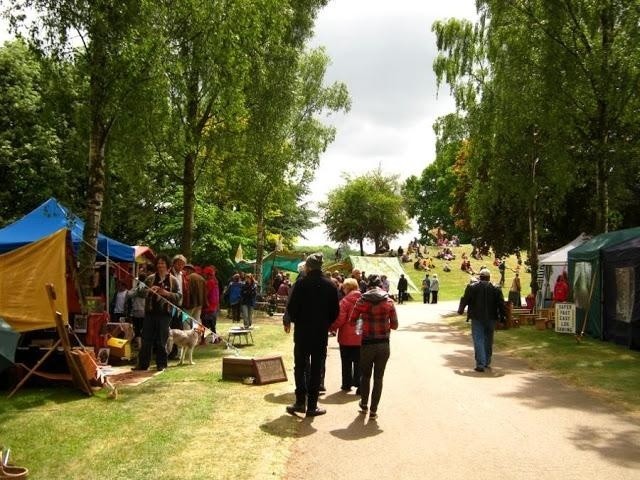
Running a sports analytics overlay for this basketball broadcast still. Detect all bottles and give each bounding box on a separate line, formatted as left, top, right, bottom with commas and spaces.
356, 314, 364, 336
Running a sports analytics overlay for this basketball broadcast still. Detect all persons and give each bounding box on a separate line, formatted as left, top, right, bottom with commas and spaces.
273, 271, 291, 296
335, 248, 341, 259
379, 232, 531, 284
113, 253, 219, 371
223, 273, 257, 329
512, 274, 521, 290
458, 269, 506, 371
395, 274, 439, 303
282, 253, 399, 418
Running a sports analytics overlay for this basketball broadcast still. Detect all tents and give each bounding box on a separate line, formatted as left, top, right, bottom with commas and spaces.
225, 253, 305, 289
568, 227, 640, 351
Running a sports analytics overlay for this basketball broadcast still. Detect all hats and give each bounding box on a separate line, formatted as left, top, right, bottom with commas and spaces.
306, 253, 324, 270
480, 269, 491, 277
366, 274, 383, 286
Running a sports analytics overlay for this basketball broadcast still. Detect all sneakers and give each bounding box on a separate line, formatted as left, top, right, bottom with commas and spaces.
341, 386, 351, 390
306, 409, 326, 416
475, 367, 484, 372
131, 366, 146, 370
359, 402, 367, 410
287, 406, 305, 413
369, 411, 376, 420
158, 367, 163, 370
319, 384, 326, 391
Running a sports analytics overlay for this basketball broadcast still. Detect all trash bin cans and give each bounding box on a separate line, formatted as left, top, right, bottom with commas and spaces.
496, 300, 513, 330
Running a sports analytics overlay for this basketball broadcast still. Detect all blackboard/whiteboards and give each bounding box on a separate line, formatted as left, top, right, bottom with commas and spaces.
555, 303, 577, 333
252, 355, 288, 385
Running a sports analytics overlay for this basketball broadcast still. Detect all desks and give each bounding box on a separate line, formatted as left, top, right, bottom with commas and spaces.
513, 308, 539, 325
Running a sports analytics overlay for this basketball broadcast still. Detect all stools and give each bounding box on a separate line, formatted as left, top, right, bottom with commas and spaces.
228, 332, 254, 346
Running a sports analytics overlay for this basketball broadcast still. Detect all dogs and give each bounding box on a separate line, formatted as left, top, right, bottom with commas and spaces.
165, 319, 205, 366
